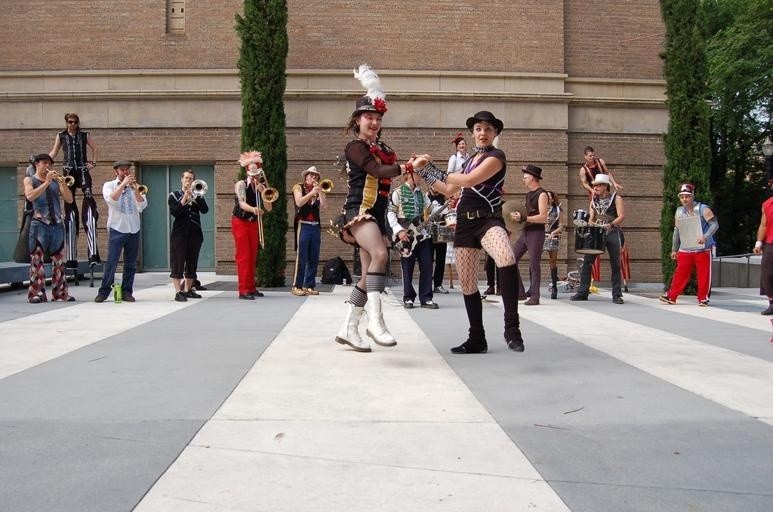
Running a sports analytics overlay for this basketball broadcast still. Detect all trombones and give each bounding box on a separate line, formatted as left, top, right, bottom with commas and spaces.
254, 170, 279, 249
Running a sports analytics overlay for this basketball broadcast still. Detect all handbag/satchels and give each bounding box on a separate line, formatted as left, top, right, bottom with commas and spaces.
323, 256, 352, 284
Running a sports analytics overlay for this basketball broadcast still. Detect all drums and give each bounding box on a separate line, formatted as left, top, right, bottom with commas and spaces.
543, 238, 559, 250
433, 226, 454, 242
572, 209, 590, 227
574, 227, 607, 255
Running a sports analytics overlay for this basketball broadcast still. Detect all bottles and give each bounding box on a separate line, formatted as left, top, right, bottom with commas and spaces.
111, 282, 122, 303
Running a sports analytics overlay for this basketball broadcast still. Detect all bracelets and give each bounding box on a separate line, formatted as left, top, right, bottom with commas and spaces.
755, 241, 762, 248
521, 214, 527, 222
609, 222, 614, 228
405, 163, 413, 174
92, 163, 96, 167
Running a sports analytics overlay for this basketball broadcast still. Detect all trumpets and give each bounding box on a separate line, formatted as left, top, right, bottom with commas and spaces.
124, 174, 148, 195
313, 179, 334, 194
46, 168, 75, 187
186, 179, 208, 199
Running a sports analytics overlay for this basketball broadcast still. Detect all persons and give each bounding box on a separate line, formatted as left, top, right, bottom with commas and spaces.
292, 165, 328, 296
526, 191, 563, 299
168, 170, 209, 303
485, 255, 501, 295
659, 184, 720, 307
231, 162, 272, 300
411, 110, 525, 353
23, 154, 76, 303
753, 180, 773, 315
94, 160, 148, 303
333, 96, 431, 353
579, 146, 624, 202
49, 113, 102, 265
510, 164, 549, 305
570, 174, 624, 304
387, 136, 470, 309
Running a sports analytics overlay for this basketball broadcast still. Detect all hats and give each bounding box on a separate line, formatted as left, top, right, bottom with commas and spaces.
677, 184, 694, 195
521, 164, 543, 179
350, 96, 387, 118
33, 154, 53, 163
237, 149, 263, 176
590, 173, 612, 187
302, 166, 321, 181
112, 160, 132, 168
465, 111, 503, 136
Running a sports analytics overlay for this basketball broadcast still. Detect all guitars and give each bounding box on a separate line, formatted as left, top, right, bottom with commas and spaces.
391, 198, 454, 258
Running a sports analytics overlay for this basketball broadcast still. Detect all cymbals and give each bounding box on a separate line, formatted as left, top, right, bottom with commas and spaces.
502, 201, 528, 230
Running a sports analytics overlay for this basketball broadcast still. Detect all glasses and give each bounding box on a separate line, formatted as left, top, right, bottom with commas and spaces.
66, 120, 78, 125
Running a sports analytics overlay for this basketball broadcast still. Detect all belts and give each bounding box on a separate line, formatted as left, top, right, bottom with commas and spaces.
461, 210, 503, 220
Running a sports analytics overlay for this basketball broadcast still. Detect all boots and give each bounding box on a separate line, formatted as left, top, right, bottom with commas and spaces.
363, 293, 396, 346
526, 267, 531, 297
450, 290, 487, 354
334, 304, 371, 352
550, 268, 557, 299
496, 262, 524, 351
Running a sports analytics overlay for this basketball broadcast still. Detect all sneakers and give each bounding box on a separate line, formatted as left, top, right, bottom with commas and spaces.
484, 285, 540, 305
402, 299, 413, 308
66, 295, 74, 301
122, 295, 135, 301
433, 285, 448, 294
659, 295, 675, 304
761, 309, 773, 315
699, 301, 708, 307
95, 293, 106, 302
175, 292, 187, 302
184, 290, 201, 298
240, 291, 263, 299
292, 288, 318, 295
31, 295, 41, 302
420, 300, 438, 309
612, 295, 623, 303
569, 293, 588, 300
